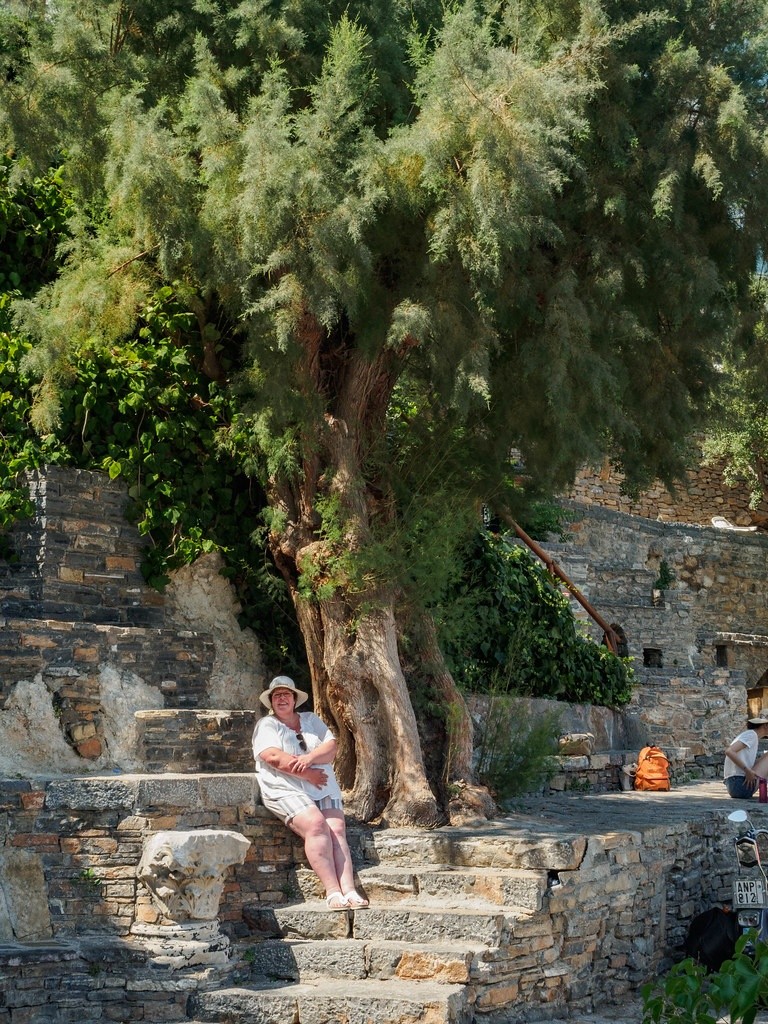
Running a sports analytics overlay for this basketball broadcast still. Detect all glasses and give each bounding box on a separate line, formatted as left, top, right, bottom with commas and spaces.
270, 690, 294, 698
296, 733, 307, 752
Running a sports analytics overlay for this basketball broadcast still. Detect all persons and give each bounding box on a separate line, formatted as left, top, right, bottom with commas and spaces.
252, 676, 369, 911
723, 709, 768, 799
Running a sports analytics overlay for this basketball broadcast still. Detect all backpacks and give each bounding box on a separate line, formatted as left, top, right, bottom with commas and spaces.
633, 741, 672, 791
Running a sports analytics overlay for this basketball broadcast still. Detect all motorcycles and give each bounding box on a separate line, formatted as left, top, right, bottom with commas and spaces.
727, 808, 768, 963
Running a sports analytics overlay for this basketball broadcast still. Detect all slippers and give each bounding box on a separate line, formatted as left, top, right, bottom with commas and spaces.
326, 892, 351, 911
344, 891, 368, 909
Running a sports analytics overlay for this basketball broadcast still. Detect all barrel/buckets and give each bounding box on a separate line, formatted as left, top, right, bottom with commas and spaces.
619, 769, 637, 792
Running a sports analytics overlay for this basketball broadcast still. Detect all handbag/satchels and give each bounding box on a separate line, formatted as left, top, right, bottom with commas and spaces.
559, 732, 597, 756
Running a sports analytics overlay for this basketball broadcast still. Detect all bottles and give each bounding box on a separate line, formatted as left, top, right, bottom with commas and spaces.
759, 778, 767, 803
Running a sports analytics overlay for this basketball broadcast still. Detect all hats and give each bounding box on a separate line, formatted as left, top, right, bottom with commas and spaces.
748, 709, 768, 724
258, 676, 308, 710
